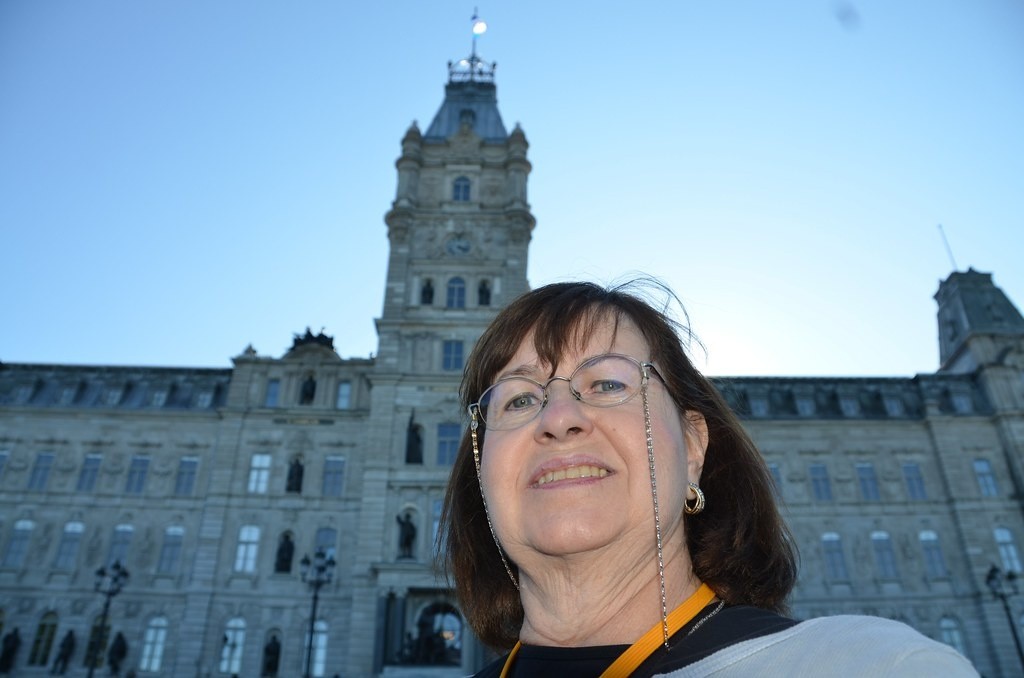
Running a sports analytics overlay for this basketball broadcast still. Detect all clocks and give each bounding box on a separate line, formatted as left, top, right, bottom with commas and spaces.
445, 235, 471, 257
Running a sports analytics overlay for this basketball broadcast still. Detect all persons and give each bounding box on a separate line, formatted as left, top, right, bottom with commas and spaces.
480, 283, 490, 305
288, 459, 303, 491
278, 535, 292, 572
0, 627, 21, 673
303, 376, 315, 401
106, 633, 126, 678
422, 281, 433, 303
432, 282, 984, 678
263, 636, 279, 678
406, 417, 422, 463
396, 514, 415, 558
51, 631, 75, 674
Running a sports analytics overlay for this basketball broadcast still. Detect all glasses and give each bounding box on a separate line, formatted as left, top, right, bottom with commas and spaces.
467, 353, 686, 430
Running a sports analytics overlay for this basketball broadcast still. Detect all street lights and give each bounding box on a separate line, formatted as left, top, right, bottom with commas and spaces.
987, 565, 1024, 667
302, 546, 336, 678
86, 559, 131, 678
